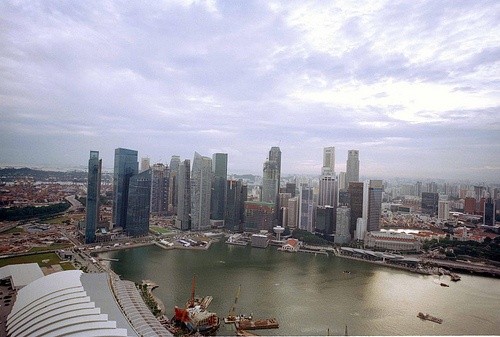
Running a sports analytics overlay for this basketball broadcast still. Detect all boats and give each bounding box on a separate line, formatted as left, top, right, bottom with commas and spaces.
181, 273, 221, 335
417, 312, 442, 324
450, 273, 461, 281
342, 271, 351, 274
224, 313, 279, 330
440, 283, 449, 287
